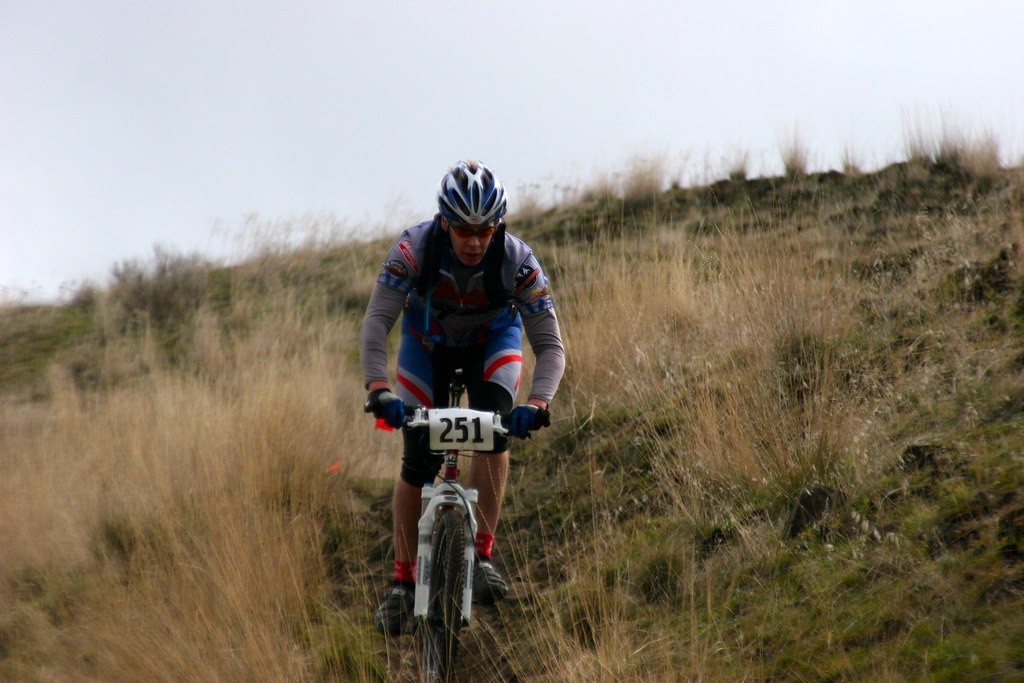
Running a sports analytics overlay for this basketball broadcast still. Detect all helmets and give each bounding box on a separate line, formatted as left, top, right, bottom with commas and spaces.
437, 160, 507, 227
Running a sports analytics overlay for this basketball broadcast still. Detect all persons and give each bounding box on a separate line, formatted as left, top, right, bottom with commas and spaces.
359, 160, 566, 637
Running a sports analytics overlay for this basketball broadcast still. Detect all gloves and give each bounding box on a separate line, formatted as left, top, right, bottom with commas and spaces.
507, 403, 551, 440
367, 389, 406, 431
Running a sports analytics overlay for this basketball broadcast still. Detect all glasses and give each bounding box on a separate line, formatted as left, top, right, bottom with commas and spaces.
445, 217, 499, 238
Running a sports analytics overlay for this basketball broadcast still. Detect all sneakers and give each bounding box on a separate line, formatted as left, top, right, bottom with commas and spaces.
374, 585, 416, 638
472, 561, 508, 600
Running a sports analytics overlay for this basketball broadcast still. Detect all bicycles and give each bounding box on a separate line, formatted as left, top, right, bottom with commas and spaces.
363, 366, 533, 683
